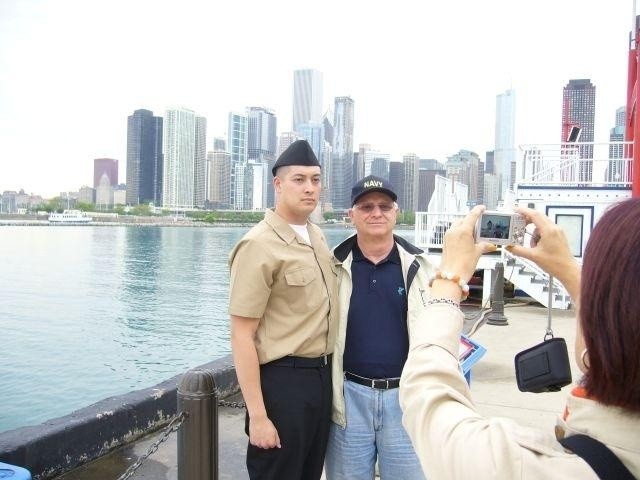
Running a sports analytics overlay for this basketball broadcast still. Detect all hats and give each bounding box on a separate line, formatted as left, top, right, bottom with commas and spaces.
351, 175, 398, 207
271, 139, 321, 178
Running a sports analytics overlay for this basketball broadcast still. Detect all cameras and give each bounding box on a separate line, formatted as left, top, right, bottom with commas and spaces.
474, 210, 525, 246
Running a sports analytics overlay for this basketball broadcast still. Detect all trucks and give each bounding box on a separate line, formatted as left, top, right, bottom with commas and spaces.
18, 208, 31, 214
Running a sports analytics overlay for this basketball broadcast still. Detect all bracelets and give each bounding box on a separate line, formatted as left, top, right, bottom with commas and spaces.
428, 269, 470, 303
427, 298, 462, 310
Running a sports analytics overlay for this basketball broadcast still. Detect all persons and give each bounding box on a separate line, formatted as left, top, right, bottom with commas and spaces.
324, 175, 440, 480
400, 198, 640, 480
493, 224, 504, 238
228, 141, 338, 480
480, 220, 494, 238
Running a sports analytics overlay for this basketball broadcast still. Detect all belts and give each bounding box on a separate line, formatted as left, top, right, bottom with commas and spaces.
345, 371, 398, 390
270, 353, 331, 369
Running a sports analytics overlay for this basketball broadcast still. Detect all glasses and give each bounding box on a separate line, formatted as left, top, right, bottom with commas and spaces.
353, 201, 399, 213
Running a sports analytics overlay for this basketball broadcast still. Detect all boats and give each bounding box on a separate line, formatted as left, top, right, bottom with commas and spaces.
48, 208, 93, 224
